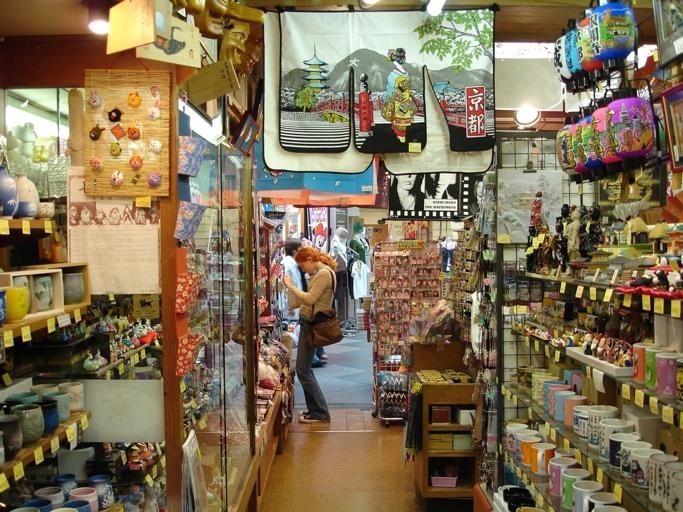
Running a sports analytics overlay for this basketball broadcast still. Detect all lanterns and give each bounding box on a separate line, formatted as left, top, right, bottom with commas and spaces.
552, 0, 658, 183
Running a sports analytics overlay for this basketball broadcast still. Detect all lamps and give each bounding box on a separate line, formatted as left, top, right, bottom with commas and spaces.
81, 0, 111, 36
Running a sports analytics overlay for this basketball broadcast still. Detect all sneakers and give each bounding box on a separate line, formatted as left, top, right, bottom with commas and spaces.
298, 410, 323, 423
312, 353, 327, 367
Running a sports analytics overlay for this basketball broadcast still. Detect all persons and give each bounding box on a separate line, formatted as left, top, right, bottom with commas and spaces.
377, 46, 413, 144
425, 173, 455, 199
526, 192, 602, 275
388, 172, 427, 211
330, 227, 355, 332
282, 246, 338, 424
278, 237, 328, 368
348, 222, 370, 266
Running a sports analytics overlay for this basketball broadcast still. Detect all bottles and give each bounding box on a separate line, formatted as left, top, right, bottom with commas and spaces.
14, 172, 40, 219
53, 316, 154, 371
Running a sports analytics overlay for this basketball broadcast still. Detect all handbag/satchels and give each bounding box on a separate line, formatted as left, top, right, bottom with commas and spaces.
310, 316, 344, 348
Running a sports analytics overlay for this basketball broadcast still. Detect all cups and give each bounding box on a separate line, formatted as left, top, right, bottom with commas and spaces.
9, 473, 115, 512
0, 272, 86, 327
0, 382, 85, 464
633, 340, 682, 404
510, 356, 682, 511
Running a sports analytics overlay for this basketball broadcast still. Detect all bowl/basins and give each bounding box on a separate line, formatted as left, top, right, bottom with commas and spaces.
35, 202, 54, 221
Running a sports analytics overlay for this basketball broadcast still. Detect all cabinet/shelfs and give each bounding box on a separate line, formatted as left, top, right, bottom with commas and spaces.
496, 258, 682, 512
406, 341, 478, 498
0, 219, 92, 492
